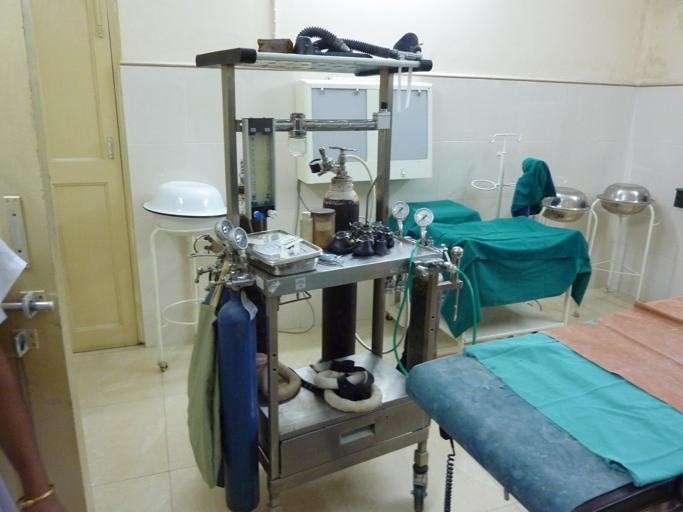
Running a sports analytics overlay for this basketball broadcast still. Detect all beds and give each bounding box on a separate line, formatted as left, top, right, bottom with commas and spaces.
402, 299, 681, 512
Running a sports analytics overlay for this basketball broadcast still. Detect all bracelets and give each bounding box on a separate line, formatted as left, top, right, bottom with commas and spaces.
15, 484, 58, 509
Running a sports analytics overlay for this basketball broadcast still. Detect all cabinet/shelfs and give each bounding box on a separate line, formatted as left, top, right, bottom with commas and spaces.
195, 49, 434, 511
295, 79, 432, 186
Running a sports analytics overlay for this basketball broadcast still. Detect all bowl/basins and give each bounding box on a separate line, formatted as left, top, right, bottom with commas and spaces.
596, 183, 652, 216
539, 186, 591, 223
142, 180, 228, 219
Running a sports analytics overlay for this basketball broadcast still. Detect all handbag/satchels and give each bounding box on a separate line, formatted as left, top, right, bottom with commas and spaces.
187, 258, 230, 490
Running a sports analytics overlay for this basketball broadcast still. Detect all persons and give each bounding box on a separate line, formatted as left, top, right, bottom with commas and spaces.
0, 239, 63, 512
